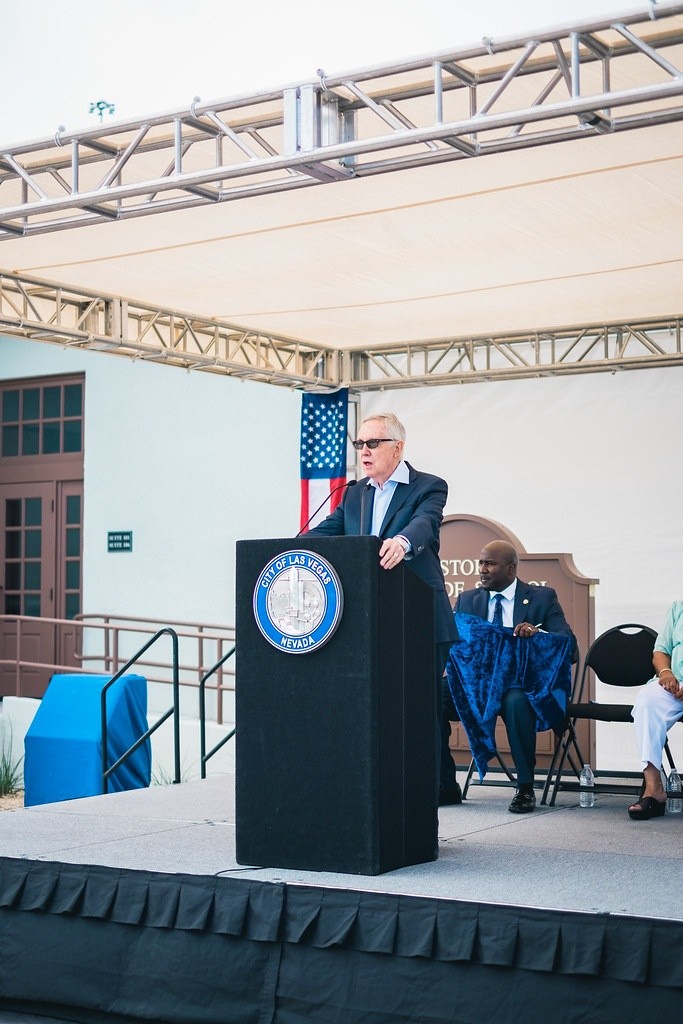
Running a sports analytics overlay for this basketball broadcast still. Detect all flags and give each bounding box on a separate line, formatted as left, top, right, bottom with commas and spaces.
299, 386, 349, 535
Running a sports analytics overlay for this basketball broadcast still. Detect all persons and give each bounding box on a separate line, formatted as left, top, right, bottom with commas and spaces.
438, 541, 578, 813
628, 599, 683, 821
300, 411, 458, 681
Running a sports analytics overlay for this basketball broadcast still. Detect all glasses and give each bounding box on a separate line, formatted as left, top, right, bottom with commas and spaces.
352, 438, 400, 450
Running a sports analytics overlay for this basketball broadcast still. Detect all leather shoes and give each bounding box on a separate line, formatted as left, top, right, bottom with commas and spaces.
509, 789, 536, 813
438, 783, 462, 806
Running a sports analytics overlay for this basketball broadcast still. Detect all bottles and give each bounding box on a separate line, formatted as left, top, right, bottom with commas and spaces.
580, 764, 594, 808
667, 769, 682, 812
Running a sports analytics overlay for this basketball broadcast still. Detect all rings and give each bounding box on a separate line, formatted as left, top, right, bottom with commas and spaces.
527, 626, 533, 632
393, 552, 399, 558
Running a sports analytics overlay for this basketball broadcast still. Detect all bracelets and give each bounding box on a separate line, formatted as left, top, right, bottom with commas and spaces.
659, 669, 673, 677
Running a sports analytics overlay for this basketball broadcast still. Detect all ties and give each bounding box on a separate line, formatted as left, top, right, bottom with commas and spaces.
492, 594, 504, 626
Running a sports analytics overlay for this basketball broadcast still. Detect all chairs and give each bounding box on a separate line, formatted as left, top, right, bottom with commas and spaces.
462, 622, 683, 807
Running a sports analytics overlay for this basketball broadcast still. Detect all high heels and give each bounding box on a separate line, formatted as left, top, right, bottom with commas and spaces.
627, 797, 667, 821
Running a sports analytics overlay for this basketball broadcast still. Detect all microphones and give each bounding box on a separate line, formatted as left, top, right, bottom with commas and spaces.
295, 479, 357, 538
